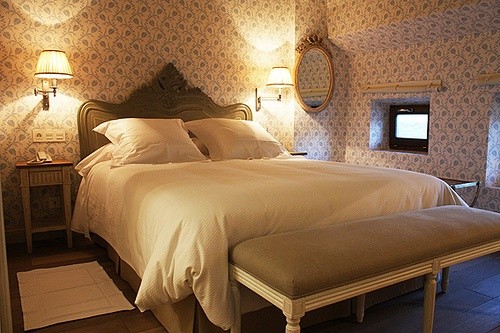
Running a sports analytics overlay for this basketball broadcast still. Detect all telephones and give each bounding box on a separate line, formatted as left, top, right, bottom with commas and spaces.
26, 150, 53, 163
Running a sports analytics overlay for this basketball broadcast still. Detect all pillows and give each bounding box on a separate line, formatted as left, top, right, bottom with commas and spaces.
182, 118, 292, 161
92, 118, 209, 167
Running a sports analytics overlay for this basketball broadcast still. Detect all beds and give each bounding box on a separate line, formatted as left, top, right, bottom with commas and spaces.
71, 62, 469, 333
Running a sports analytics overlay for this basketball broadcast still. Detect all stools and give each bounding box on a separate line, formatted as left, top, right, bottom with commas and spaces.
439, 178, 481, 208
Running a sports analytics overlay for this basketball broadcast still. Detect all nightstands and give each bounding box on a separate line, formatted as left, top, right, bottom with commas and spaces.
16, 160, 79, 251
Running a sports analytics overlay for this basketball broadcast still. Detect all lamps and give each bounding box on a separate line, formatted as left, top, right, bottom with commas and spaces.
33, 50, 74, 111
255, 67, 294, 111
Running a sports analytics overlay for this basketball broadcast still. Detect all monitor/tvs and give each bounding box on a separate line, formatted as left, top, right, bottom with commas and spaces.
389, 105, 429, 152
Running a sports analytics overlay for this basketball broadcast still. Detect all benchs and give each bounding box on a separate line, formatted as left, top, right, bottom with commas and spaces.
229, 204, 500, 333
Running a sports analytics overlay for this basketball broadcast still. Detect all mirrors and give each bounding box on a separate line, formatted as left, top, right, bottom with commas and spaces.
294, 34, 334, 113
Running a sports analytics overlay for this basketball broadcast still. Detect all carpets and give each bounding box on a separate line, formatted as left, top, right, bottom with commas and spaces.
17, 261, 136, 330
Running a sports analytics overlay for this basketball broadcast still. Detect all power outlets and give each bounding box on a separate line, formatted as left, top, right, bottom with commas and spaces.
33, 129, 45, 142
